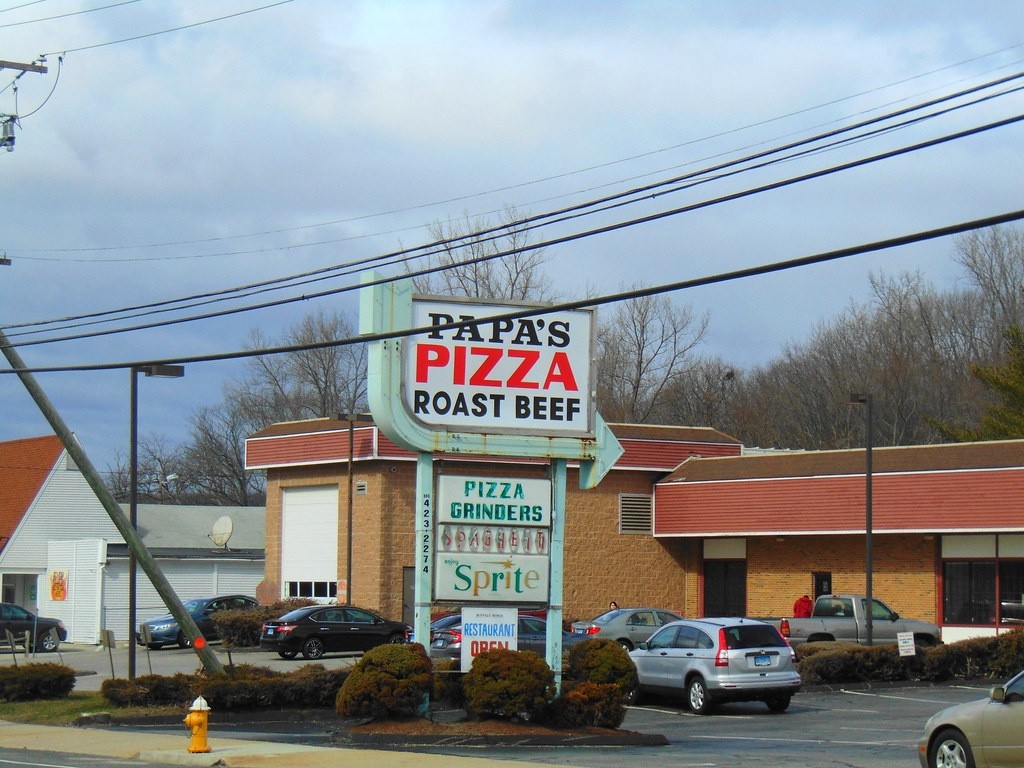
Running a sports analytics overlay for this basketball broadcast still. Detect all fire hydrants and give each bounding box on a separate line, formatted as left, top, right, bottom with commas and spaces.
183, 695, 212, 753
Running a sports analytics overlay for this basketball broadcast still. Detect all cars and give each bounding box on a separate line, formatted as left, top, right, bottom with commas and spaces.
137, 594, 261, 651
404, 615, 462, 647
428, 614, 594, 660
572, 608, 684, 653
916, 666, 1024, 768
261, 604, 408, 660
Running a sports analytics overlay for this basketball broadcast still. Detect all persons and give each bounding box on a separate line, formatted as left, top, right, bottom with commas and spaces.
792, 596, 813, 619
609, 602, 618, 612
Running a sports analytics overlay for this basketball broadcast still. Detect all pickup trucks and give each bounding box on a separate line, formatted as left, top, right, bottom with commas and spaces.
754, 596, 943, 654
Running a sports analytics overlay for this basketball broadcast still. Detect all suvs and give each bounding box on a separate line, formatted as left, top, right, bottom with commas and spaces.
0, 603, 67, 652
629, 616, 802, 715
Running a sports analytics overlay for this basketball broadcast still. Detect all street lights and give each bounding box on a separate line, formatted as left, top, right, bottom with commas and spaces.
849, 390, 873, 648
126, 359, 185, 683
